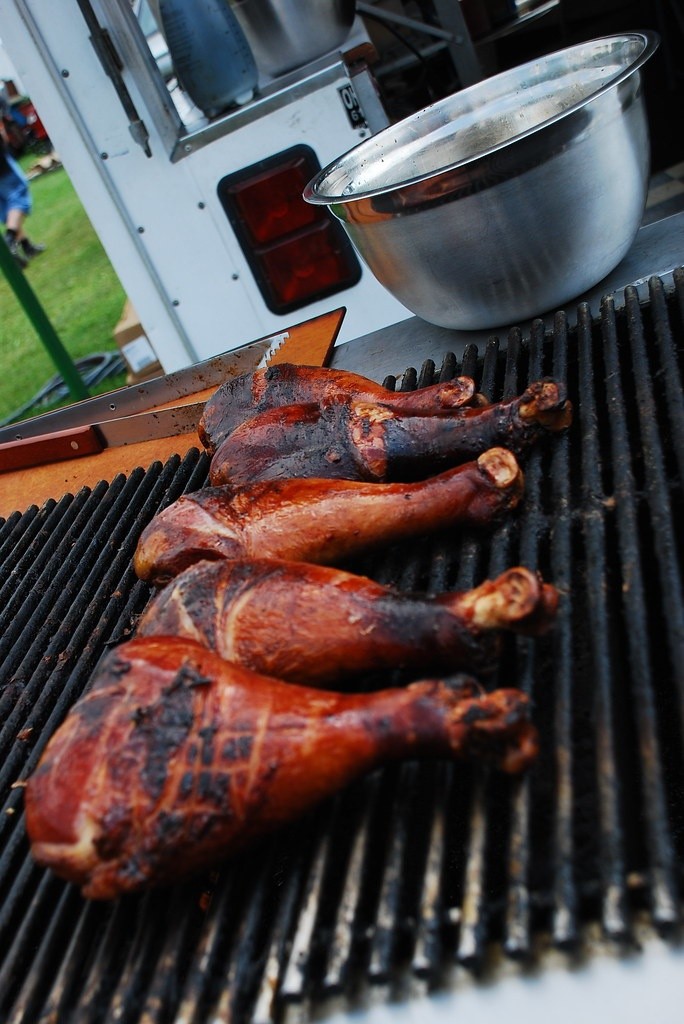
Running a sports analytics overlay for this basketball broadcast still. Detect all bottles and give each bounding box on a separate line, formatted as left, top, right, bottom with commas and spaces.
158, 1, 259, 121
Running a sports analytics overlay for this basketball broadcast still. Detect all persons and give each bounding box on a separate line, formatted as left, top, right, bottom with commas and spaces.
0, 80, 46, 270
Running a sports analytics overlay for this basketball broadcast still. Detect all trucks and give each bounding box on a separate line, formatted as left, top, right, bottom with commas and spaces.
0, 0, 684, 390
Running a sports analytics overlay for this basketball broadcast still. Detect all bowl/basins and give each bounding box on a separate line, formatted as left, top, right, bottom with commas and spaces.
303, 32, 660, 331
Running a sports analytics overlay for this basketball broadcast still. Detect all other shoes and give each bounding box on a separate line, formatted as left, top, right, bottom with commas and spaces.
25, 244, 45, 258
4, 238, 27, 265
